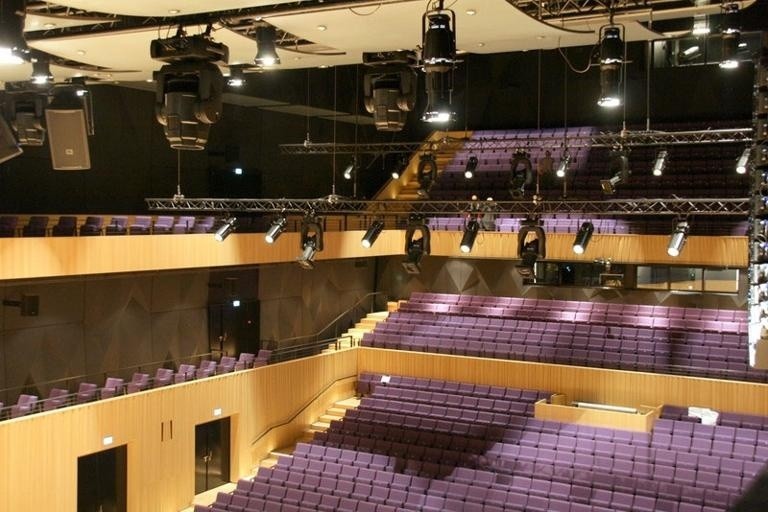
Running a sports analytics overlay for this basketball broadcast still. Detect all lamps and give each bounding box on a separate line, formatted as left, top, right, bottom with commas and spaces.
356, 4, 465, 134
679, 3, 740, 76
509, 217, 554, 279
343, 161, 361, 180
662, 220, 694, 259
390, 157, 412, 182
212, 213, 241, 244
456, 222, 482, 255
298, 213, 329, 268
571, 218, 597, 256
262, 210, 293, 245
2, 5, 89, 98
361, 218, 387, 250
584, 12, 634, 118
399, 215, 434, 277
143, 9, 281, 157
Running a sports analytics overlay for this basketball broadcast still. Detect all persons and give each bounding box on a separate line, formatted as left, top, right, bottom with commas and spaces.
459, 194, 481, 226
536, 149, 557, 189
479, 197, 504, 231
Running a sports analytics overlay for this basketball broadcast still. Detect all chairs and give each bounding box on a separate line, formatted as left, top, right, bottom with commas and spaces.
408, 121, 752, 236
193, 292, 766, 512
0, 364, 196, 424
3, 214, 214, 237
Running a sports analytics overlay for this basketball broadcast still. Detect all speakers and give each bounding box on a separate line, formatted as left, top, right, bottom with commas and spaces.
44, 89, 95, 171
0, 112, 23, 164
